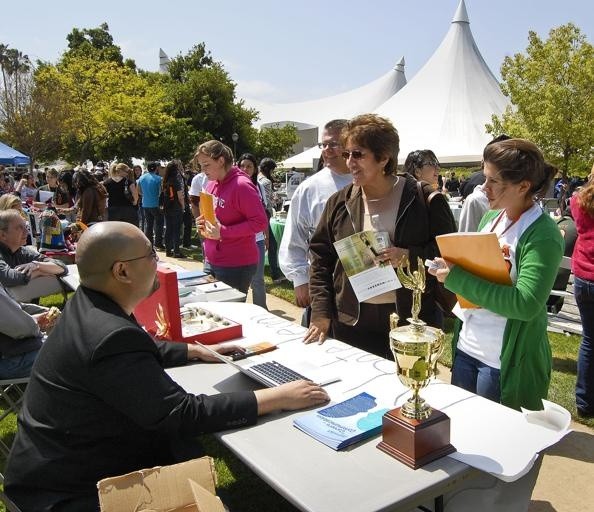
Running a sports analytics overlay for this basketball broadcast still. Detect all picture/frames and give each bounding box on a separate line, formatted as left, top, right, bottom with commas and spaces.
264, 214, 286, 265
140, 300, 550, 512
56, 258, 246, 299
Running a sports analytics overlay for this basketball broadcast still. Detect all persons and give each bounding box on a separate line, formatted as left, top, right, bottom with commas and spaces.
554, 169, 579, 204
0, 192, 23, 225
257, 157, 281, 283
1, 224, 329, 512
2, 209, 69, 284
195, 141, 267, 302
569, 158, 594, 418
131, 165, 142, 182
440, 172, 451, 193
84, 159, 120, 183
428, 139, 564, 412
99, 162, 139, 220
460, 136, 511, 239
189, 155, 220, 226
52, 172, 76, 216
547, 207, 577, 312
74, 168, 110, 227
305, 115, 455, 350
1, 288, 60, 376
445, 174, 458, 194
161, 159, 187, 258
0, 170, 25, 195
237, 154, 266, 207
403, 149, 439, 185
34, 166, 69, 208
21, 173, 35, 198
137, 162, 167, 249
278, 120, 356, 325
288, 168, 296, 182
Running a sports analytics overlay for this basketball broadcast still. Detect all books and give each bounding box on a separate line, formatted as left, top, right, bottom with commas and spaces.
96, 456, 224, 512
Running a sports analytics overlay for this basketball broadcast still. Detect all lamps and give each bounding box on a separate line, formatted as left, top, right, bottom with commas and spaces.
0, 378, 30, 420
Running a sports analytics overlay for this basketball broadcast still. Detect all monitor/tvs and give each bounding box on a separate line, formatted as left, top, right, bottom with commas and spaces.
434, 233, 511, 308
291, 392, 395, 452
197, 186, 215, 230
334, 229, 403, 301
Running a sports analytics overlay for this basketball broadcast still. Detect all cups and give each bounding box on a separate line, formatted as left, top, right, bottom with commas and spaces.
316, 141, 341, 150
421, 160, 441, 168
111, 248, 159, 271
341, 150, 371, 159
117, 168, 129, 174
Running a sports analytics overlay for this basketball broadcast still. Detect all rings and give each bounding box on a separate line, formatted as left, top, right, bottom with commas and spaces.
363, 238, 385, 265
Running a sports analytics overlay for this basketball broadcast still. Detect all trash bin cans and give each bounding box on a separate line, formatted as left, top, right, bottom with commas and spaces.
31, 259, 41, 276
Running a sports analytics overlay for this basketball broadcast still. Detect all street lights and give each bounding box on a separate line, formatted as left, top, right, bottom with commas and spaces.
257, 181, 271, 222
414, 180, 457, 318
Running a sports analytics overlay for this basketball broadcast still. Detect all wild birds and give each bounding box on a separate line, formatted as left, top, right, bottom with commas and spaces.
192, 344, 342, 388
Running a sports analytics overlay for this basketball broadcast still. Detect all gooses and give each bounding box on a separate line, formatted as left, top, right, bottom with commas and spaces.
375, 257, 457, 468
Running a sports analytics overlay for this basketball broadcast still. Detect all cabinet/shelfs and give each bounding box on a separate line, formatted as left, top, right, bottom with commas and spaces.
370, 215, 393, 250
